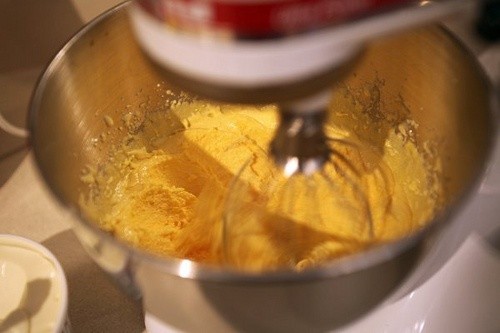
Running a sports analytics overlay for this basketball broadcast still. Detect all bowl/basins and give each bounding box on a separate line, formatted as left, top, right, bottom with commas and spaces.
28, 0, 500, 333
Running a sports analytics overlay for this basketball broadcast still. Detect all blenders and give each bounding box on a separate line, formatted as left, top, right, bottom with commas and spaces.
127, 0, 500, 272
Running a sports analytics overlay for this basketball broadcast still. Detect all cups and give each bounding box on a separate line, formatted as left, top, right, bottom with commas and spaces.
0, 236, 73, 333
